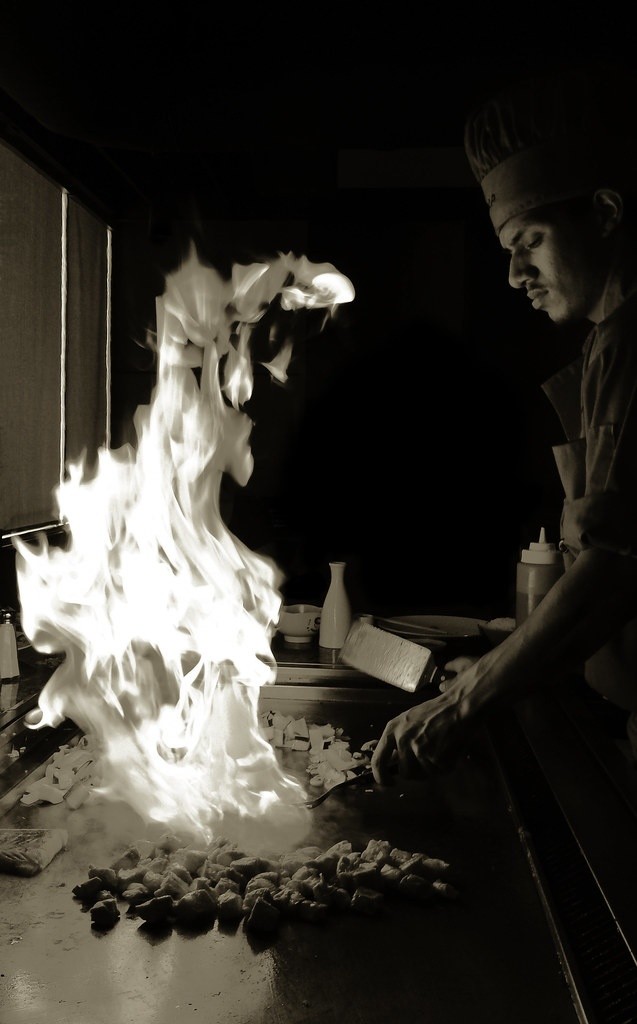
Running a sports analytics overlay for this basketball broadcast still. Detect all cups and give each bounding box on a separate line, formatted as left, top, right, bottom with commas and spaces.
353, 614, 374, 626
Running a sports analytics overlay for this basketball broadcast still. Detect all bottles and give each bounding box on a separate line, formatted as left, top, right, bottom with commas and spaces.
320, 562, 351, 648
0, 608, 20, 712
516, 527, 558, 630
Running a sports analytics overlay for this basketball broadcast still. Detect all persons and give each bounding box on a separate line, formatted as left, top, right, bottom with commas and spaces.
371, 92, 637, 786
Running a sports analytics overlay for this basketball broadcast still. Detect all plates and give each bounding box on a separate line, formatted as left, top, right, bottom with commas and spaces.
376, 615, 489, 639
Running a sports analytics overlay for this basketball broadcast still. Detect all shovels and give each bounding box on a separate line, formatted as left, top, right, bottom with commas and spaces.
338, 619, 456, 696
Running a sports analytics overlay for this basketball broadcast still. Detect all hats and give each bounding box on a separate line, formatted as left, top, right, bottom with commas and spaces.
464, 77, 637, 237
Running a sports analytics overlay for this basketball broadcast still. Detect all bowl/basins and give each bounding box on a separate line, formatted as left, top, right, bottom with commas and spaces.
478, 621, 516, 647
275, 605, 322, 642
